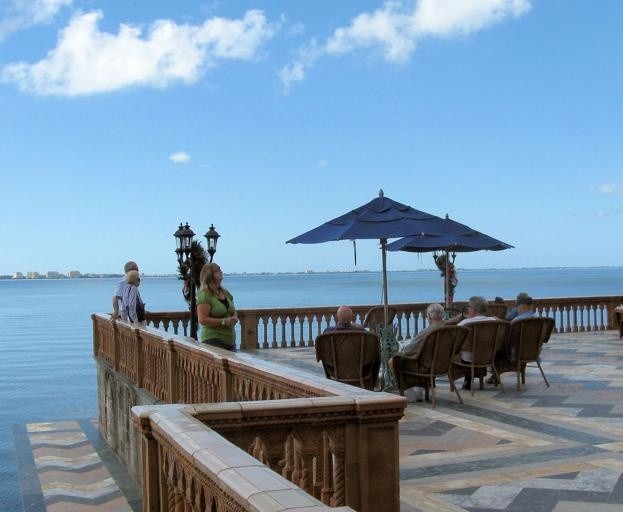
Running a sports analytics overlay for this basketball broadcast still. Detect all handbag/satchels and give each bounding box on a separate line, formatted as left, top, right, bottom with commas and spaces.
126, 303, 145, 323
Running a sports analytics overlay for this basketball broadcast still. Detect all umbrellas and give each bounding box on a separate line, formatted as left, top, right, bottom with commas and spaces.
285, 189, 474, 326
380, 213, 515, 309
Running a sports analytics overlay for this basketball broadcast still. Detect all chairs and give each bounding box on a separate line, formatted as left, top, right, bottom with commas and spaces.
316, 305, 555, 409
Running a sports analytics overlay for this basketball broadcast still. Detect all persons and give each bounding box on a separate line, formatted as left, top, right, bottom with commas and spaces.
110, 261, 138, 321
323, 305, 367, 333
196, 263, 238, 352
387, 294, 538, 402
122, 270, 146, 326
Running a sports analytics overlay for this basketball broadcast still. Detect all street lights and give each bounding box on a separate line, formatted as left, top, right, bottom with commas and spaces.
173, 221, 221, 340
432, 250, 457, 308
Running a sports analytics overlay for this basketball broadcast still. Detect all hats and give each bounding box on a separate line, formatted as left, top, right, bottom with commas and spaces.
516, 296, 532, 306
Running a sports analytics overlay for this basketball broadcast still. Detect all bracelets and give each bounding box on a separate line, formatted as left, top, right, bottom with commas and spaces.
221, 318, 226, 327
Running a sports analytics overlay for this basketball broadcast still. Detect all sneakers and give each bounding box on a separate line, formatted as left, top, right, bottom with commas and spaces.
405, 390, 423, 403
460, 383, 471, 390
485, 375, 499, 384
